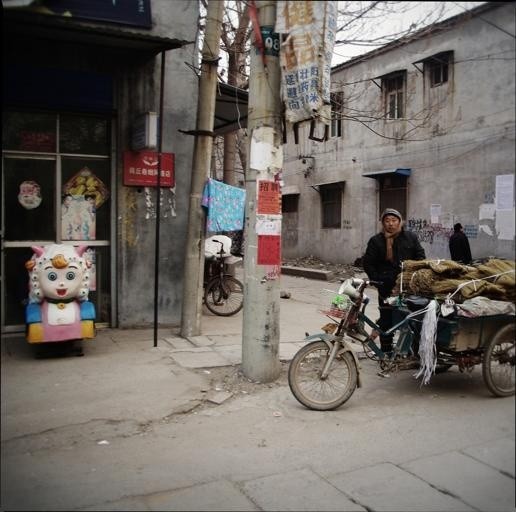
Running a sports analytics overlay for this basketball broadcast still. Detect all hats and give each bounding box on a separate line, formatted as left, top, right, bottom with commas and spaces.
381, 208, 402, 223
454, 223, 462, 231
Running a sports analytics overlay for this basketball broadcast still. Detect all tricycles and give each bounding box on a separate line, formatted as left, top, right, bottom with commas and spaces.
288, 274, 515, 412
203, 238, 244, 316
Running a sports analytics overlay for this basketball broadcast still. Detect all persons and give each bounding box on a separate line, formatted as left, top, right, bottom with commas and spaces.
449, 223, 472, 265
363, 208, 426, 352
